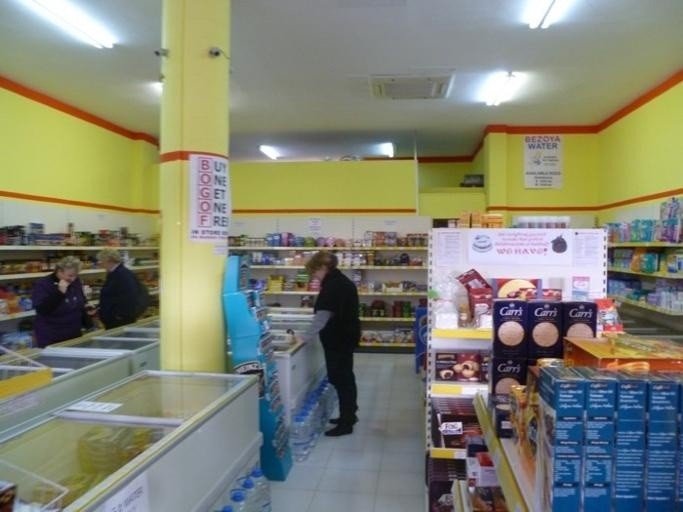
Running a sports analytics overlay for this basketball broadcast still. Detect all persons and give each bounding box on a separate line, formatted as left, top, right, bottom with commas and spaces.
290, 251, 362, 438
95, 246, 151, 332
30, 256, 96, 349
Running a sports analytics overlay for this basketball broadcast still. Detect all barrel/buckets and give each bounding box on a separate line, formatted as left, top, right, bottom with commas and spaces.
213, 465, 272, 511
288, 374, 340, 464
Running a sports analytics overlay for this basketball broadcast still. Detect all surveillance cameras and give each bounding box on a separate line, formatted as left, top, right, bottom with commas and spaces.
154, 48, 167, 56
209, 47, 221, 57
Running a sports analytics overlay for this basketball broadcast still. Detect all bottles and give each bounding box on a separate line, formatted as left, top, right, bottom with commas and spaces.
218, 467, 272, 512
287, 374, 340, 464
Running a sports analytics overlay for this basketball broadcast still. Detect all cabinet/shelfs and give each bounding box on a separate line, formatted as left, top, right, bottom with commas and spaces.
608, 243, 683, 321
1, 245, 163, 321
229, 247, 429, 348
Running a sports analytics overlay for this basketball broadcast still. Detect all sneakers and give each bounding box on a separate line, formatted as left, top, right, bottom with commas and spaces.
329, 419, 338, 424
324, 425, 353, 436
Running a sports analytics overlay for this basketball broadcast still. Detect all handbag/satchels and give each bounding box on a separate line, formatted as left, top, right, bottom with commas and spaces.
132, 284, 149, 320
